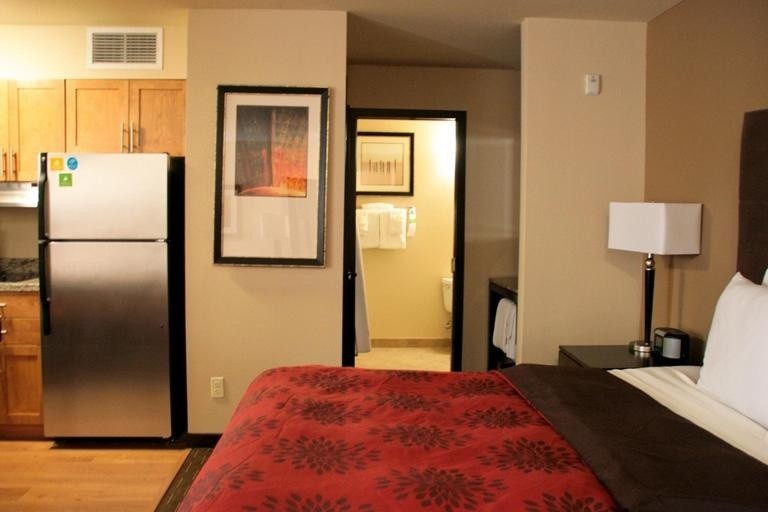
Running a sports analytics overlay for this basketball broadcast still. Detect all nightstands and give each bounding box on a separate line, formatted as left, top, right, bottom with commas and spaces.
558, 344, 705, 370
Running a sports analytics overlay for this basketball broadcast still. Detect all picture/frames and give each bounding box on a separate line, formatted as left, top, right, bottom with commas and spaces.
213, 85, 330, 266
356, 132, 415, 198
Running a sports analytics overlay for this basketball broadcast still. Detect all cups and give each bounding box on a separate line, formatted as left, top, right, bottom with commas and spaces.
663, 339, 680, 359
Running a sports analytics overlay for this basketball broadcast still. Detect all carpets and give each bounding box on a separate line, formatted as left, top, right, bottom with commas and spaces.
153, 448, 215, 512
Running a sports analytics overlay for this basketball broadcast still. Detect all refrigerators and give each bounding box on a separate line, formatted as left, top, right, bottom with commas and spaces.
38, 152, 178, 444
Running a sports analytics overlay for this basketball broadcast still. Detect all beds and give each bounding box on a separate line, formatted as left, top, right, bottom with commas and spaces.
180, 365, 767, 512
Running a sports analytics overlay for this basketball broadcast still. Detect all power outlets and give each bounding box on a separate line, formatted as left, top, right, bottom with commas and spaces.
210, 376, 224, 399
586, 73, 603, 95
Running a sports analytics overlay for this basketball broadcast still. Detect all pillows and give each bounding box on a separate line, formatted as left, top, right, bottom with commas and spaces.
697, 271, 768, 429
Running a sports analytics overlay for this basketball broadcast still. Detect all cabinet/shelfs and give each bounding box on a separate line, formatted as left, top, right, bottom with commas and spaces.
65, 79, 189, 154
0, 275, 43, 437
486, 277, 516, 369
3, 82, 64, 180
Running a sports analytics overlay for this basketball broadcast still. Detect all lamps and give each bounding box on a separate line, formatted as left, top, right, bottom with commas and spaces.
608, 202, 705, 349
